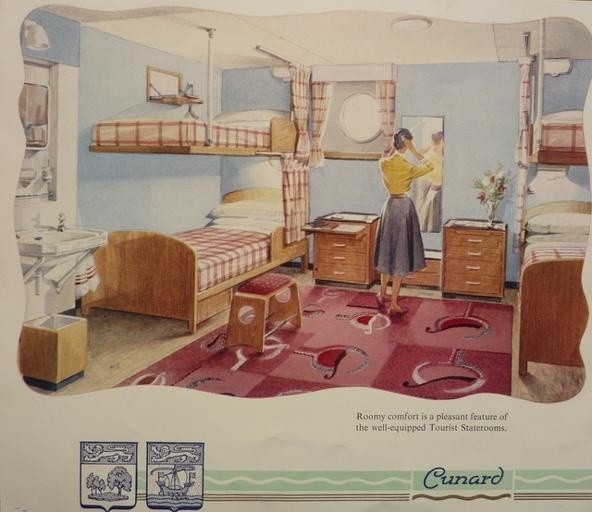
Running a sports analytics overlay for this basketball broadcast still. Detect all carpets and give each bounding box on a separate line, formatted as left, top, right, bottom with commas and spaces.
113, 284, 513, 395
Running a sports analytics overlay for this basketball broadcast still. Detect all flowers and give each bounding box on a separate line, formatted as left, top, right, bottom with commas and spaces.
473, 162, 516, 220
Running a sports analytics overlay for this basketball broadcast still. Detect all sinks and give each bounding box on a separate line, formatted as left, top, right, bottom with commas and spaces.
16, 229, 108, 257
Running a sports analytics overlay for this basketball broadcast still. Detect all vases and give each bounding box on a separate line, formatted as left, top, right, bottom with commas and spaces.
486, 200, 499, 227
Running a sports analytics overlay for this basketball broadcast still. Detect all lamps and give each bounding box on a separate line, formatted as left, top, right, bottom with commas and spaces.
23, 20, 50, 51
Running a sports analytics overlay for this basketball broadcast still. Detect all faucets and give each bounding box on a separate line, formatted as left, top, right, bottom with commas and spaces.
58, 212, 65, 228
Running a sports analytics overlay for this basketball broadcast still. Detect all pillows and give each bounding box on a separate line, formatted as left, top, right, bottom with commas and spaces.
215, 108, 291, 132
541, 109, 583, 123
208, 200, 285, 237
522, 211, 591, 244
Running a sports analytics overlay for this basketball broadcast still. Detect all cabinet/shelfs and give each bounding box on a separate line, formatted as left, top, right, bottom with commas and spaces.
440, 218, 507, 302
302, 209, 380, 290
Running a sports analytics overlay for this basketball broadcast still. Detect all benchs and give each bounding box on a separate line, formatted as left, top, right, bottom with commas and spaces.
225, 271, 303, 353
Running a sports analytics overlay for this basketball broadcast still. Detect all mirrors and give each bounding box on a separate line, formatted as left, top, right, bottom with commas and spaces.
398, 115, 443, 234
19, 82, 51, 150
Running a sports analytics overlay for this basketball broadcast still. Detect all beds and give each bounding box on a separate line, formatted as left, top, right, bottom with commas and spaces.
79, 120, 309, 336
517, 121, 589, 376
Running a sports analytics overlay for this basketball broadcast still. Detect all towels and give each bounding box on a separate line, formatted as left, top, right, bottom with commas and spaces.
76, 252, 101, 299
